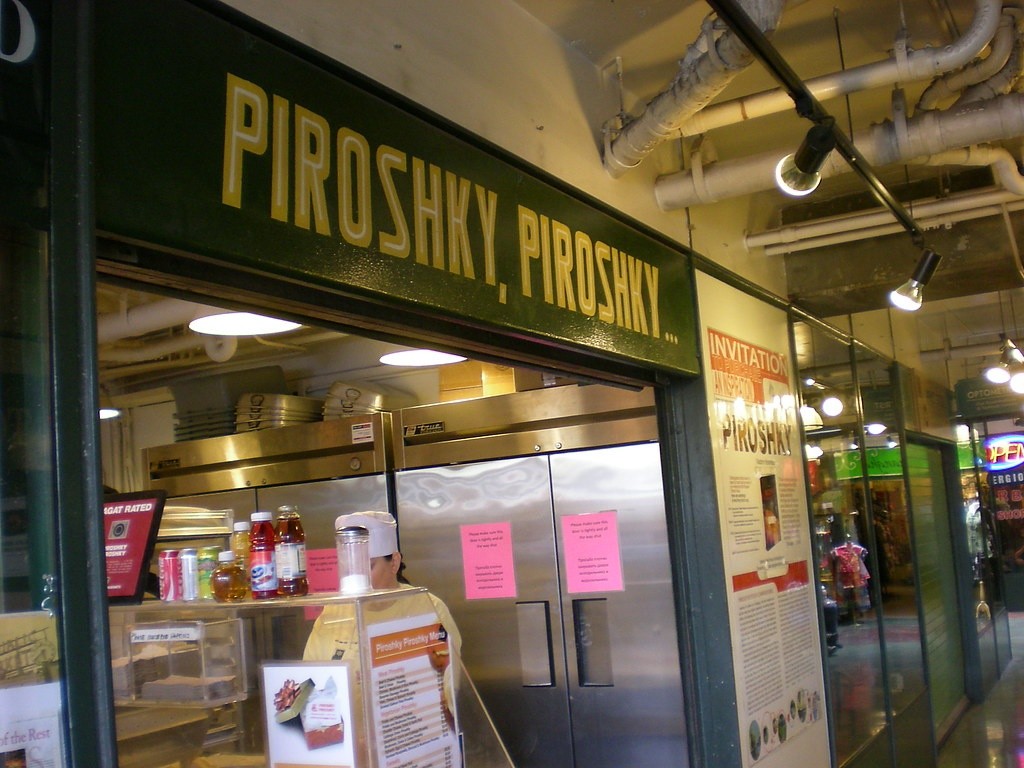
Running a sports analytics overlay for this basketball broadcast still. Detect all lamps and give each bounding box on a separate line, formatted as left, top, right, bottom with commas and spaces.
986, 348, 1016, 384
889, 247, 943, 311
774, 112, 837, 197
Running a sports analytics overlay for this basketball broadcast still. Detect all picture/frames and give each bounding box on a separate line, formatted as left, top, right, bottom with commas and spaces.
103, 488, 168, 604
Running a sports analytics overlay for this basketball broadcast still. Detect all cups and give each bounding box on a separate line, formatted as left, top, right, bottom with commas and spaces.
335, 526, 373, 596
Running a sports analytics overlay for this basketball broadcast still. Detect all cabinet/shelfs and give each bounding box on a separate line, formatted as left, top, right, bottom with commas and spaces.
110, 509, 514, 768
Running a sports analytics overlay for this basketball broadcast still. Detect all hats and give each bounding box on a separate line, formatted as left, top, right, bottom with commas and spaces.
334, 512, 399, 558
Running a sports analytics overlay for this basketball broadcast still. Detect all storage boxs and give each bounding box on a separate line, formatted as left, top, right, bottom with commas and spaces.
437, 357, 576, 402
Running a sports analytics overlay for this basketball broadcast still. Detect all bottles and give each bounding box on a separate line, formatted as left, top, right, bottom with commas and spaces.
274, 505, 308, 599
230, 521, 251, 601
210, 550, 247, 603
249, 511, 278, 601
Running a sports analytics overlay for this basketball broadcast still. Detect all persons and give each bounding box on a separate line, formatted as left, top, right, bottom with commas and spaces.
301, 510, 462, 714
